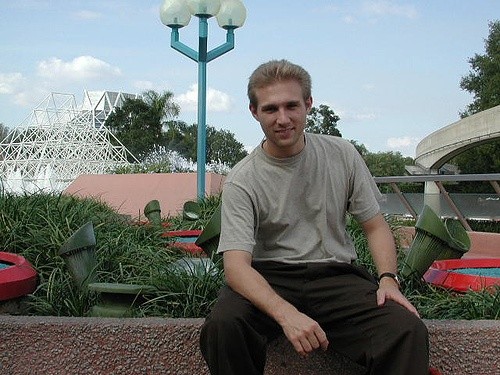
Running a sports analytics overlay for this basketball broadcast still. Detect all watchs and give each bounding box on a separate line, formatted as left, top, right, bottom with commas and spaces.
377, 272, 401, 288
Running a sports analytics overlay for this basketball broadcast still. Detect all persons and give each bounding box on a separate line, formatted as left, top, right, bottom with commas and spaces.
200, 59, 430, 375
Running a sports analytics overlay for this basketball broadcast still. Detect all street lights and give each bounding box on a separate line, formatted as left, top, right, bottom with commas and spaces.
158, 0, 249, 206
